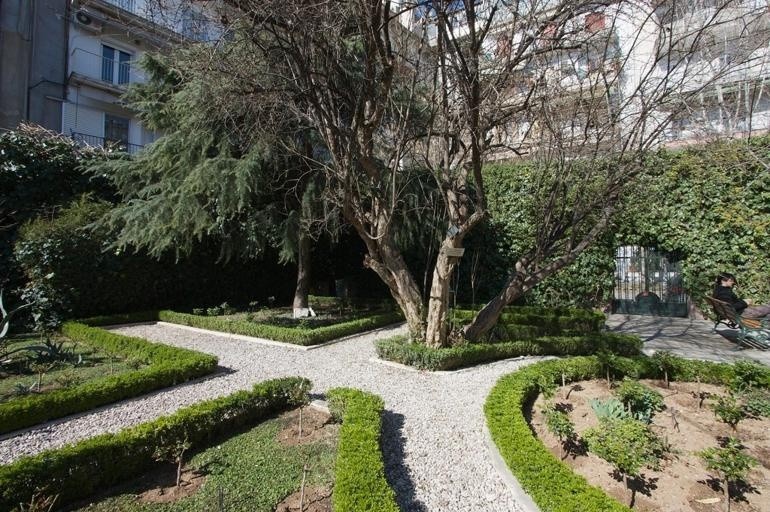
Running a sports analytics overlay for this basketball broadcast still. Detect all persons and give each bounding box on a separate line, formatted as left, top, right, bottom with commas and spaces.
712, 271, 770, 321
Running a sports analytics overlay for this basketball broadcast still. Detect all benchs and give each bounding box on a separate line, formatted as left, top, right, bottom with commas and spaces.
701, 294, 770, 350
